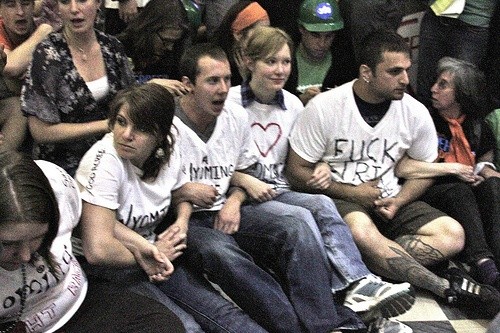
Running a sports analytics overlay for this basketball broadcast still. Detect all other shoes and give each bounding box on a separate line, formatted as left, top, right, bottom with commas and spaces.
481, 260, 500, 285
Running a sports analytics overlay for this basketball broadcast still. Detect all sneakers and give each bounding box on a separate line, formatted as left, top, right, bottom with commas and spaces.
343, 273, 415, 318
366, 319, 413, 333
445, 267, 500, 319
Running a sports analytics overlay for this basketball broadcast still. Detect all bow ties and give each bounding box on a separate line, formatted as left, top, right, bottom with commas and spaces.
430, 0, 466, 18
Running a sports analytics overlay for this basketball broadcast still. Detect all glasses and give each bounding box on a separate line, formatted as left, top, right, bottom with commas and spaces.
305, 30, 330, 39
437, 79, 456, 92
157, 32, 181, 47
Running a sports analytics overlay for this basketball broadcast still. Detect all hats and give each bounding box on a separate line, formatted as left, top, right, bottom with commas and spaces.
298, 0, 344, 32
